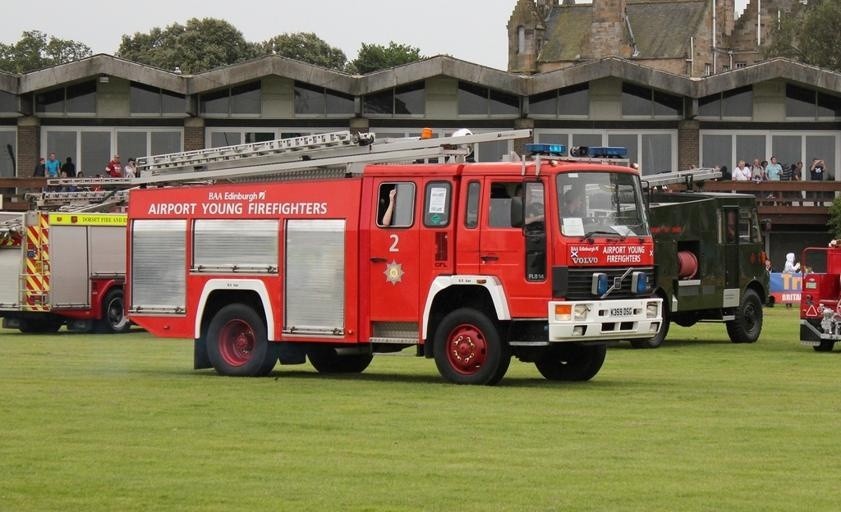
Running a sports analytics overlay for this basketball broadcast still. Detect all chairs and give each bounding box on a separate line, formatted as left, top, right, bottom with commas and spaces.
489, 187, 511, 226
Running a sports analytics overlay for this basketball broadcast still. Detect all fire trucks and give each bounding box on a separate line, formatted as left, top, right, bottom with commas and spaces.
123, 127, 667, 384
799, 240, 841, 353
602, 165, 772, 349
0, 176, 234, 335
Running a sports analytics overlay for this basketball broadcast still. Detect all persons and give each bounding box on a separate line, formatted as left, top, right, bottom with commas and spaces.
507, 183, 545, 227
381, 186, 396, 225
686, 156, 803, 207
34, 154, 150, 192
765, 252, 815, 309
810, 158, 826, 206
559, 189, 592, 225
